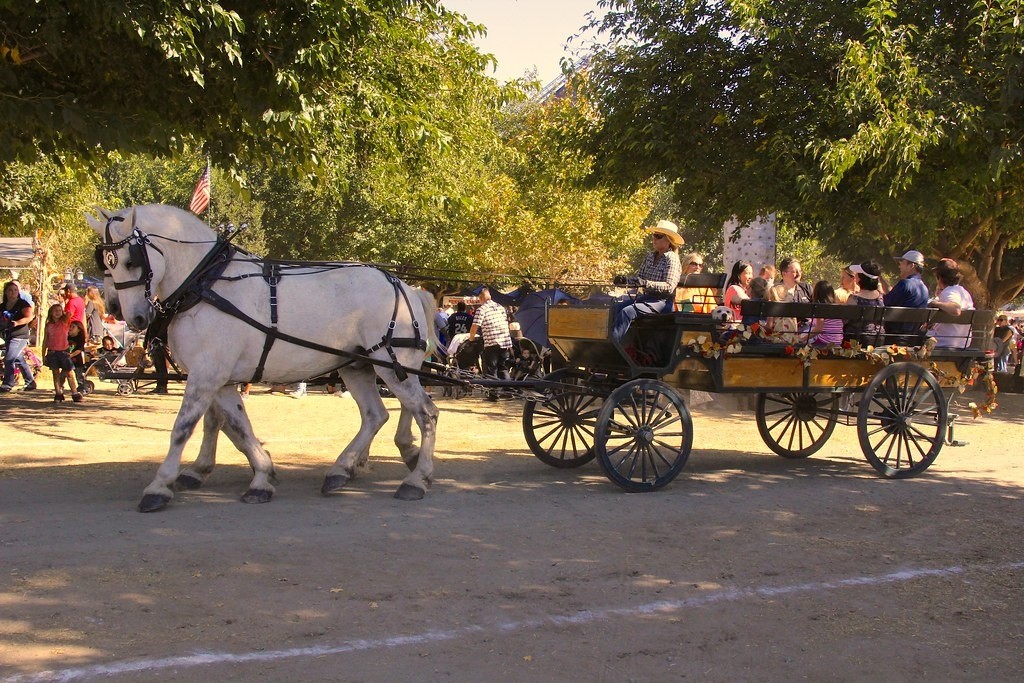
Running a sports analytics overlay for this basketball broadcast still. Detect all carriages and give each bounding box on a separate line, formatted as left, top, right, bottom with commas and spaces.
81, 203, 1000, 513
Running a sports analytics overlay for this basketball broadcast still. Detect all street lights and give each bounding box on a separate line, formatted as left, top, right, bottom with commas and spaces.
64, 267, 83, 283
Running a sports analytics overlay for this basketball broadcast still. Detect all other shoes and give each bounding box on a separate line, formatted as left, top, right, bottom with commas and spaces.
334, 391, 351, 397
54, 393, 66, 403
72, 393, 83, 402
290, 382, 307, 398
25, 380, 36, 391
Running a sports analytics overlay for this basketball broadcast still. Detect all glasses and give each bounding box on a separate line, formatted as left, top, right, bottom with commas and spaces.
690, 262, 704, 269
653, 234, 665, 239
997, 319, 1006, 322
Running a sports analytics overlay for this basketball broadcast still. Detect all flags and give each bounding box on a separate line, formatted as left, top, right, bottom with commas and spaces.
191, 163, 210, 215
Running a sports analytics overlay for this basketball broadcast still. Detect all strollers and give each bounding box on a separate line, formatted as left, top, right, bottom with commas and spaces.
81, 323, 148, 396
443, 333, 486, 399
509, 335, 551, 393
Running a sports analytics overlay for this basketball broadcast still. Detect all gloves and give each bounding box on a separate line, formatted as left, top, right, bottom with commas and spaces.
613, 275, 645, 287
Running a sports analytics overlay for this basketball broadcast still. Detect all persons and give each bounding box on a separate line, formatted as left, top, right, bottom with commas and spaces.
590, 220, 685, 362
681, 251, 1024, 373
0, 280, 571, 402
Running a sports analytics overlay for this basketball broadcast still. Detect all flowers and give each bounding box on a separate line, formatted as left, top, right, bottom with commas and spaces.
685, 320, 999, 420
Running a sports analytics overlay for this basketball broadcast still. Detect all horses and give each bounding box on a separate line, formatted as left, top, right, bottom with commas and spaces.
83, 204, 449, 512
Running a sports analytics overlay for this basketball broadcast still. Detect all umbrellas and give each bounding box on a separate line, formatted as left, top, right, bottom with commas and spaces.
512, 291, 585, 347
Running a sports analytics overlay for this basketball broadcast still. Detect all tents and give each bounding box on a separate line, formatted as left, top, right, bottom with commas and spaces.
0, 238, 34, 269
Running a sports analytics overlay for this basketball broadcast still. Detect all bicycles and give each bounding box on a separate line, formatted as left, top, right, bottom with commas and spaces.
0, 343, 20, 383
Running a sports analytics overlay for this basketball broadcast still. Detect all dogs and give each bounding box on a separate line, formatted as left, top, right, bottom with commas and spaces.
712, 306, 735, 332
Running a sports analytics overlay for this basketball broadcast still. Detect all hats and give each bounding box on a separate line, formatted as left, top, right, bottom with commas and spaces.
995, 315, 1007, 319
931, 258, 958, 272
893, 250, 924, 266
849, 264, 879, 279
645, 219, 685, 244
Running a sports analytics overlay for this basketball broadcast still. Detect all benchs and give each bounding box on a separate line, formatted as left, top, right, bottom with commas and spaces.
631, 273, 727, 328
716, 300, 993, 357
